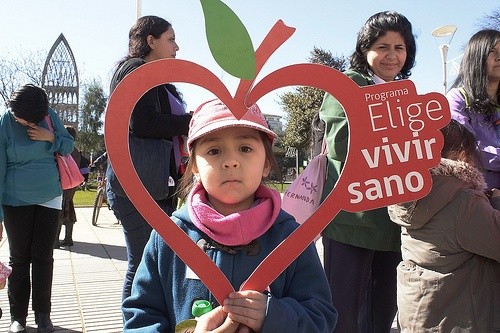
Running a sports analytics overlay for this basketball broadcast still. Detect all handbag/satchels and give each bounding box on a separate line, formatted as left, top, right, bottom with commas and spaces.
45, 115, 84, 189
281, 154, 327, 225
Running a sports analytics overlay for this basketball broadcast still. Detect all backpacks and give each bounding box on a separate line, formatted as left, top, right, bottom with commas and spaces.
79, 155, 89, 182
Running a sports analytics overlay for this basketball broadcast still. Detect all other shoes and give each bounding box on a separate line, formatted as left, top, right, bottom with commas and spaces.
53, 240, 60, 249
10, 317, 25, 333
37, 312, 53, 333
60, 236, 73, 245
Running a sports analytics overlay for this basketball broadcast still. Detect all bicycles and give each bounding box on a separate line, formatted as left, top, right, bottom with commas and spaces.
90, 163, 111, 226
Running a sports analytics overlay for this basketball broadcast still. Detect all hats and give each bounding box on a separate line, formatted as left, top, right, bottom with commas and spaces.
187, 99, 278, 155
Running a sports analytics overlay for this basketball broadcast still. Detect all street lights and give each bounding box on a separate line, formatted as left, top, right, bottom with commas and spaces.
432, 24, 457, 96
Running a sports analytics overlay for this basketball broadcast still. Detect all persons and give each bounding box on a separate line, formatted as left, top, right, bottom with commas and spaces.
105, 16, 194, 324
386, 117, 500, 333
87, 152, 107, 173
0, 83, 75, 333
317, 11, 417, 333
120, 99, 339, 333
444, 27, 500, 191
53, 126, 81, 248
